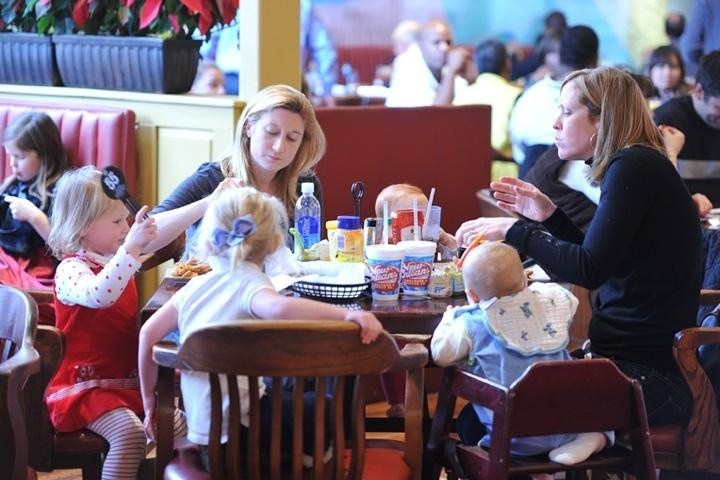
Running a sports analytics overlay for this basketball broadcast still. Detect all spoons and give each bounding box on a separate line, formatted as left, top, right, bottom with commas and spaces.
100, 165, 151, 224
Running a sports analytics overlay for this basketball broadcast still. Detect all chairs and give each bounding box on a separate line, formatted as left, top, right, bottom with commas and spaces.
0, 285, 40, 480
615, 288, 720, 479
151, 319, 401, 480
138, 230, 186, 274
20, 325, 109, 480
422, 358, 658, 479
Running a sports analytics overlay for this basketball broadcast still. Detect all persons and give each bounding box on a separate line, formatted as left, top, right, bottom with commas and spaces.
297, 0, 338, 96
0, 111, 74, 291
43, 164, 189, 479
375, 11, 720, 480
189, 61, 226, 95
137, 185, 385, 478
140, 84, 337, 396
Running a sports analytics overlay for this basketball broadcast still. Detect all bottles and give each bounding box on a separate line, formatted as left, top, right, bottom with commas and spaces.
293, 182, 322, 260
324, 207, 450, 264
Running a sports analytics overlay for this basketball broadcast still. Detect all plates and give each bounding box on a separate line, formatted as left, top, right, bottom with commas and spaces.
526, 265, 551, 284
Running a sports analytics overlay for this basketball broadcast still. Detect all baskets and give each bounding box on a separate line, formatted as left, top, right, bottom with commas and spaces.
292, 276, 372, 299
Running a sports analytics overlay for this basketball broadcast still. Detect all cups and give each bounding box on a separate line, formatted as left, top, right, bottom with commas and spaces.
366, 240, 437, 302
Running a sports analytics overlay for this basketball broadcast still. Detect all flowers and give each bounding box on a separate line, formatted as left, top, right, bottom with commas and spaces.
0, 1, 241, 41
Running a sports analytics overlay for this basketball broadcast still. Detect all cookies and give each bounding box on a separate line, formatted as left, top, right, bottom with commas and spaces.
172, 258, 212, 278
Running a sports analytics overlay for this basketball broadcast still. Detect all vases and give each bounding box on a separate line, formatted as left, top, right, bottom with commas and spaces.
49, 35, 203, 94
0, 32, 65, 87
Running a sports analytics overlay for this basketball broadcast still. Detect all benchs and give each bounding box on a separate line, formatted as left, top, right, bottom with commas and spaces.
0, 102, 138, 199
335, 45, 533, 105
